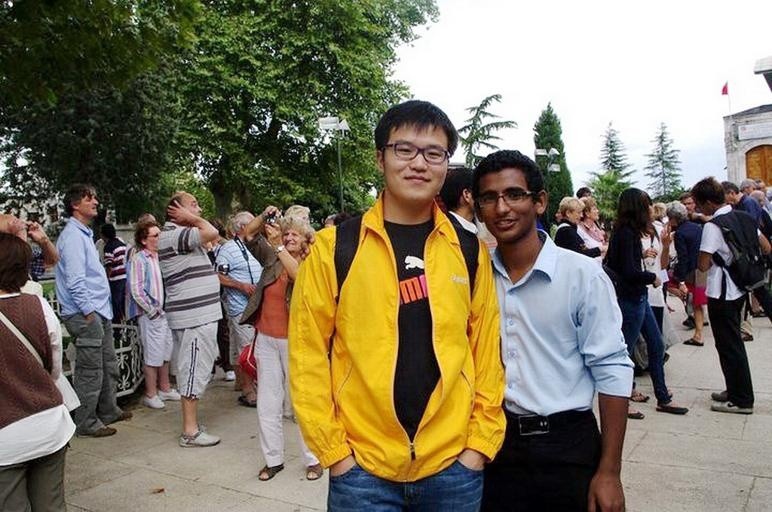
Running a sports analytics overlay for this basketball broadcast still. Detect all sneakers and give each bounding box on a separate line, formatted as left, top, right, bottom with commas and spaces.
158, 389, 181, 401
77, 425, 118, 438
224, 371, 237, 381
143, 395, 165, 409
101, 411, 133, 424
712, 390, 729, 401
711, 400, 753, 415
179, 431, 221, 447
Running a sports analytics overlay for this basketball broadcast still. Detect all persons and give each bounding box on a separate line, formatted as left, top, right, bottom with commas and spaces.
0, 179, 352, 511
295, 149, 639, 510
439, 167, 480, 234
285, 98, 509, 512
555, 178, 772, 418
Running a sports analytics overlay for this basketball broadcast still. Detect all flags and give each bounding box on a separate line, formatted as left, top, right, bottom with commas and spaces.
721, 83, 728, 96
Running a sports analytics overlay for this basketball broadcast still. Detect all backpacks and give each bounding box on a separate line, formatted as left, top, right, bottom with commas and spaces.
716, 219, 771, 294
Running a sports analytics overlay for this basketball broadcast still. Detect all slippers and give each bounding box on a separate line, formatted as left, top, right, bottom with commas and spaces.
683, 338, 705, 347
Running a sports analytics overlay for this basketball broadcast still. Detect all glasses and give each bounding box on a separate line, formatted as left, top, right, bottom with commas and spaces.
383, 138, 452, 165
476, 188, 540, 207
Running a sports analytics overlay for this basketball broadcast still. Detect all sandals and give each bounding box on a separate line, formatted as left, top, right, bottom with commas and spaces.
656, 401, 688, 414
631, 393, 650, 402
239, 392, 258, 408
306, 464, 324, 481
258, 464, 285, 481
628, 407, 645, 419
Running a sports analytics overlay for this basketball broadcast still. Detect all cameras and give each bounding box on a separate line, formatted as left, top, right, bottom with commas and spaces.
219, 263, 230, 275
266, 212, 276, 227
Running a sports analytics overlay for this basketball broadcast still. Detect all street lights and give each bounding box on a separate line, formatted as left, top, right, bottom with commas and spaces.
533, 145, 563, 228
